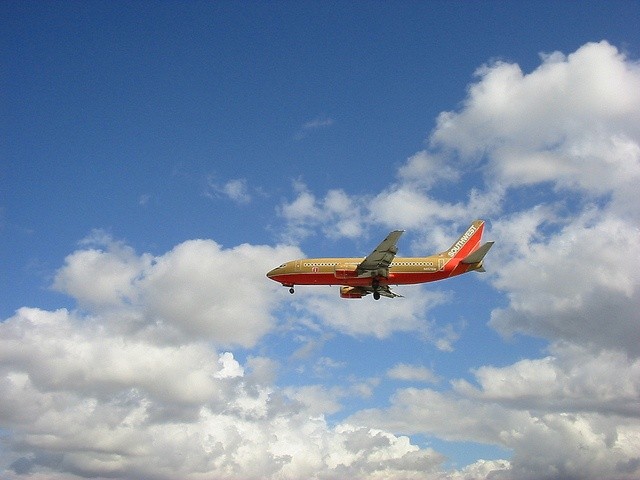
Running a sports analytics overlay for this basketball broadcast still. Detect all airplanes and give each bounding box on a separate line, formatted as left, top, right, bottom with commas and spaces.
266, 219, 496, 301
